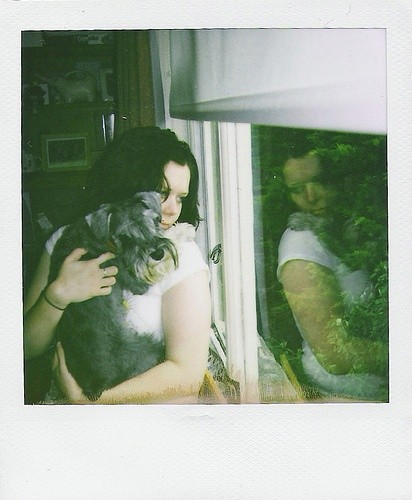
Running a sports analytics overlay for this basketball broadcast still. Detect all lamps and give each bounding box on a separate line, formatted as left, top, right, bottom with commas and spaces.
21, 55, 50, 108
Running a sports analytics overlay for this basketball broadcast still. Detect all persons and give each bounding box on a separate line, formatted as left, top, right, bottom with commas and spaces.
23, 127, 240, 403
271, 134, 390, 401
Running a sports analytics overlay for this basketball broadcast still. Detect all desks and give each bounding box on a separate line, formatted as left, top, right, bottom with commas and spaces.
22, 167, 101, 242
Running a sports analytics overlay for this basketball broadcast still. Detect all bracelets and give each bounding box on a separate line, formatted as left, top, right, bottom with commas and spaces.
41, 288, 71, 312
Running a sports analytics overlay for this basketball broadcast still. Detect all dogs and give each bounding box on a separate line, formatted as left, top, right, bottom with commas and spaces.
49, 192, 180, 403
